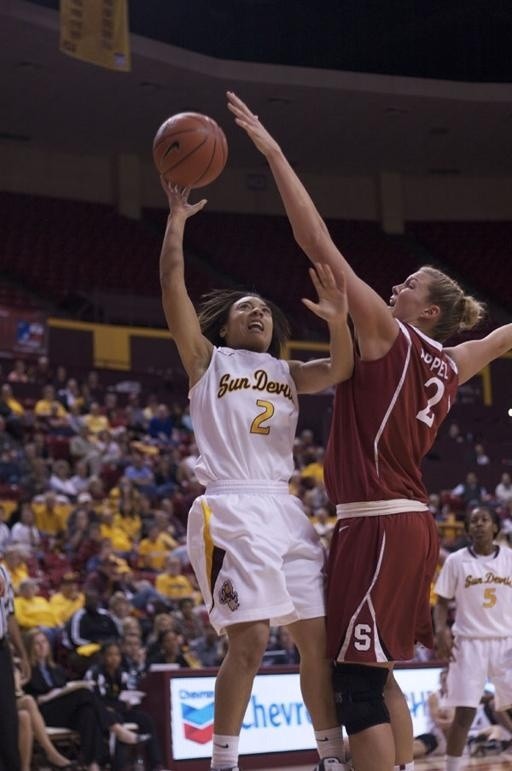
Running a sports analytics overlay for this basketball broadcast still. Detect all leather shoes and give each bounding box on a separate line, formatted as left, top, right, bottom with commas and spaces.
115, 733, 153, 748
45, 754, 77, 769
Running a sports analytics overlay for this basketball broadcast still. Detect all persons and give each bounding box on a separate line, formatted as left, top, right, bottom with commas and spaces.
226, 84, 512, 769
156, 166, 356, 771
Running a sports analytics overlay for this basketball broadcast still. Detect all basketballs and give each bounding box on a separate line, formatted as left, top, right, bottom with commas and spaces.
153, 112, 228, 189
